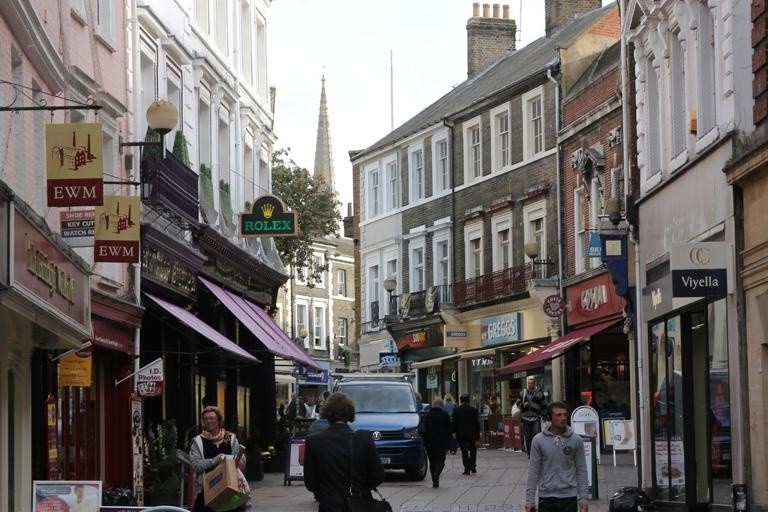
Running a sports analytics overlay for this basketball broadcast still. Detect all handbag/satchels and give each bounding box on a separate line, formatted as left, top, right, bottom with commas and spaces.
418, 422, 427, 436
512, 403, 521, 418
203, 456, 239, 510
347, 496, 393, 512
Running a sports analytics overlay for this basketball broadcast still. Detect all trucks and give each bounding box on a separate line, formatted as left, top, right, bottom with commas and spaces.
324, 370, 428, 481
654, 366, 731, 481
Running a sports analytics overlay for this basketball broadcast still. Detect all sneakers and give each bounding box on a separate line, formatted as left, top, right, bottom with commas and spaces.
471, 466, 476, 473
433, 480, 439, 487
463, 470, 470, 475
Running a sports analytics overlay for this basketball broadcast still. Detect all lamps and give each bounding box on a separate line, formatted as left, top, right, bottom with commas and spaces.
383, 277, 400, 303
524, 241, 555, 270
118, 99, 179, 161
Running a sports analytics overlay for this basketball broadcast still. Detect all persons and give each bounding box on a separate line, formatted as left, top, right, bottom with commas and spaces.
451, 393, 481, 475
523, 401, 590, 511
303, 391, 384, 511
441, 393, 458, 454
480, 398, 493, 444
188, 408, 253, 511
516, 376, 547, 460
419, 396, 449, 487
489, 396, 502, 414
67, 484, 98, 511
285, 390, 330, 421
